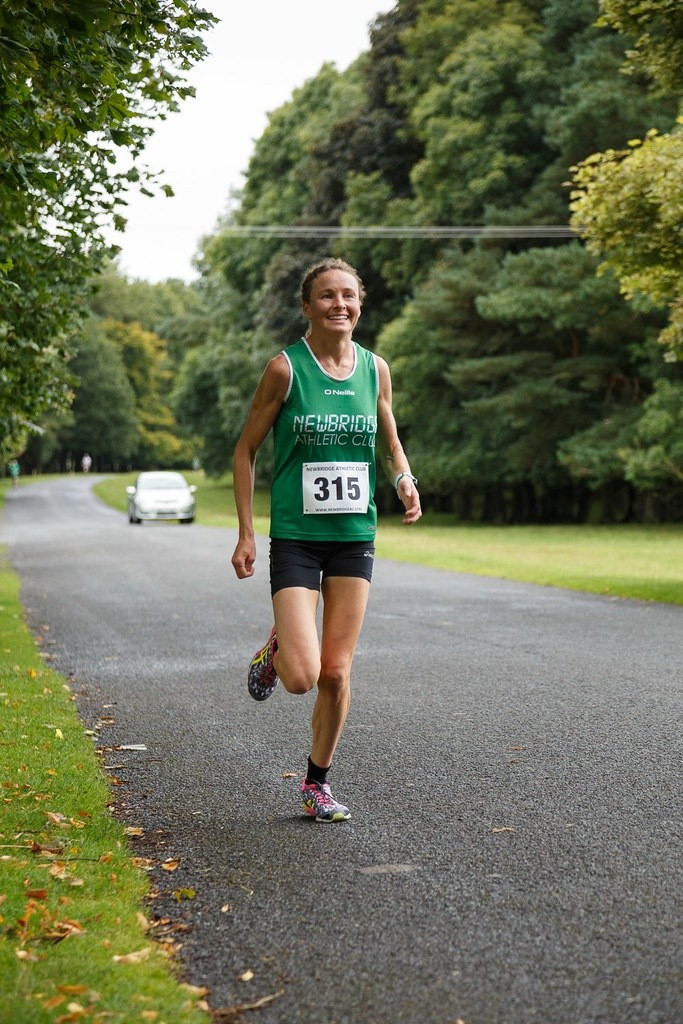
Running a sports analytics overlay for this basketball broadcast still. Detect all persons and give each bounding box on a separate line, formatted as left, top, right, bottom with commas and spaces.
9, 459, 20, 485
81, 453, 92, 473
232, 259, 423, 822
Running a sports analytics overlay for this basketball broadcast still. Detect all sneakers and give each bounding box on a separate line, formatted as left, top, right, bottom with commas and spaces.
248, 625, 280, 702
301, 776, 351, 822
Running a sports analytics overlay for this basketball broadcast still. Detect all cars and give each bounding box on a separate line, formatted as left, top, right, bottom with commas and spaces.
124, 472, 199, 524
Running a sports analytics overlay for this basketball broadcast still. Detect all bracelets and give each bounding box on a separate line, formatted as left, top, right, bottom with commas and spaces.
395, 472, 417, 500
